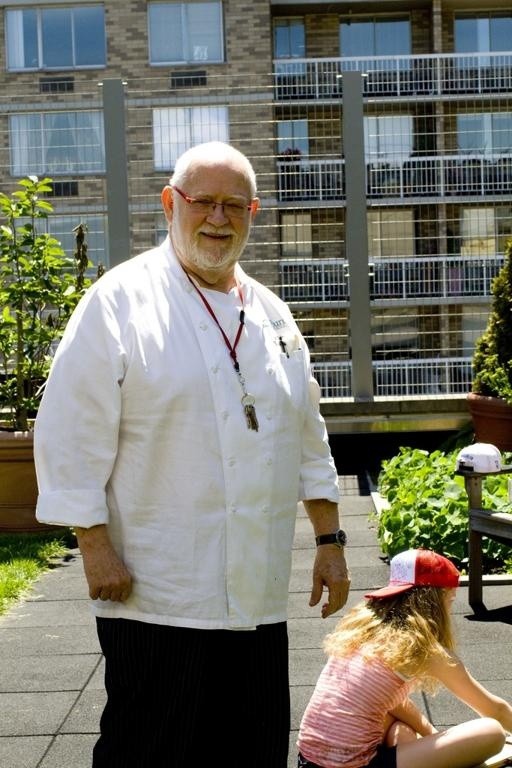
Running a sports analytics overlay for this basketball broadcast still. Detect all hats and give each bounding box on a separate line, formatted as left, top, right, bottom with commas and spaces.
364, 548, 458, 599
456, 443, 512, 473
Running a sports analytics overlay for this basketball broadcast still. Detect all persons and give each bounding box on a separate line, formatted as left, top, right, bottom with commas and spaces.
293, 548, 511, 768
31, 140, 350, 768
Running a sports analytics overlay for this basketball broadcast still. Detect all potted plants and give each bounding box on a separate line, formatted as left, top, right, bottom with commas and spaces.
469, 239, 512, 451
0, 175, 106, 532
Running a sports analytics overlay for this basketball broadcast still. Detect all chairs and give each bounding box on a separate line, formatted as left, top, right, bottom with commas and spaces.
463, 465, 512, 620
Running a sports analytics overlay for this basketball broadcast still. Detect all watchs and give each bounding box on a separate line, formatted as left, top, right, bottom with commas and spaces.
314, 529, 346, 550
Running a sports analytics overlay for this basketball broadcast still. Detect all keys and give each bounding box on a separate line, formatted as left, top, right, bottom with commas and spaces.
245, 403, 260, 433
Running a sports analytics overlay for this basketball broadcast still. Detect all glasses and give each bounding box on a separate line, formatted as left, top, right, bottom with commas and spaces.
172, 186, 251, 217
